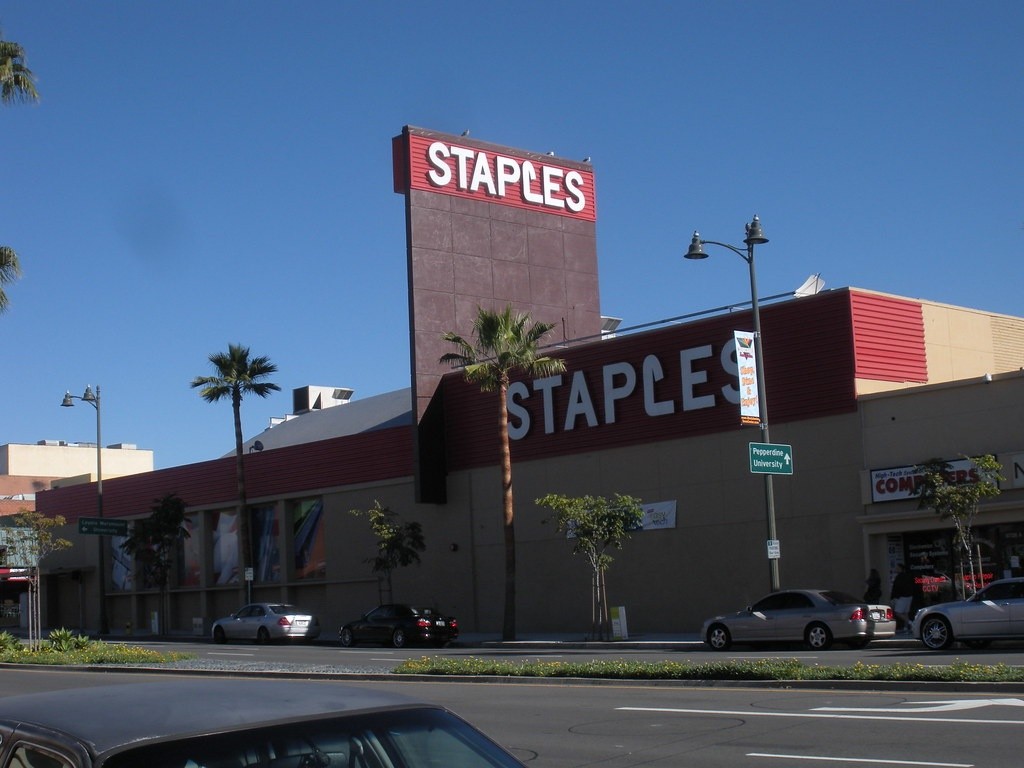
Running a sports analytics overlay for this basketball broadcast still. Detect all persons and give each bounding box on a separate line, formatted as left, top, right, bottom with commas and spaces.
863, 567, 882, 605
890, 562, 914, 634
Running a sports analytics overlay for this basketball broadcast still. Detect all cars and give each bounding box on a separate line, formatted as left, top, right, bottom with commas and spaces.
914, 578, 1024, 651
212, 603, 318, 645
340, 603, 460, 648
965, 539, 1004, 577
0, 682, 527, 768
700, 588, 897, 649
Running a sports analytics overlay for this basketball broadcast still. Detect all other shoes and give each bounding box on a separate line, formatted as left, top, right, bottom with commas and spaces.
902, 630, 909, 635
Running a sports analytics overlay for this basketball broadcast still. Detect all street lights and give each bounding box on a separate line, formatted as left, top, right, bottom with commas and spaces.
61, 383, 110, 635
684, 212, 782, 593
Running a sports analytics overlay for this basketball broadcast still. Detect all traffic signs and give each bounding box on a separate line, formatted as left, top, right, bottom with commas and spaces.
78, 516, 128, 537
749, 442, 794, 475
